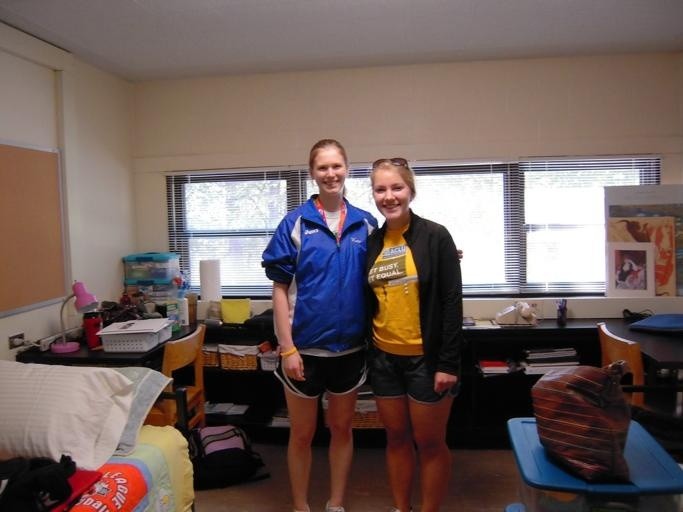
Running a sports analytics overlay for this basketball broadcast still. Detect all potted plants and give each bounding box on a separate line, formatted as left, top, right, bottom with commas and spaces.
605, 241, 656, 298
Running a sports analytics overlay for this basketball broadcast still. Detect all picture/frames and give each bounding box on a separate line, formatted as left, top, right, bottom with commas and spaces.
18, 323, 196, 401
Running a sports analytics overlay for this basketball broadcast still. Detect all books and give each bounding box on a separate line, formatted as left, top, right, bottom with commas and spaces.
507, 417, 683, 512
121, 252, 180, 279
124, 279, 178, 300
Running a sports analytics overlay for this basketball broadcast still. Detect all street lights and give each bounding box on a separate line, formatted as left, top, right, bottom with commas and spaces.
188, 425, 273, 491
531, 360, 634, 483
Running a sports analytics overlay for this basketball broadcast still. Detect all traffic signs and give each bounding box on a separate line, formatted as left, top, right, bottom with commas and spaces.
8, 332, 24, 349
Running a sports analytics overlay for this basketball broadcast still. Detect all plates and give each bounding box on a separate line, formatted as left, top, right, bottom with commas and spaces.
445, 318, 683, 450
191, 322, 386, 452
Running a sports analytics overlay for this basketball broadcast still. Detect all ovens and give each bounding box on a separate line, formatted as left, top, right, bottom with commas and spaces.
1, 387, 195, 512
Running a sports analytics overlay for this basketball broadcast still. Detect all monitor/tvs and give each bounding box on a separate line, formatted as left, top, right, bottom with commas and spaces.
479, 358, 511, 376
523, 345, 579, 375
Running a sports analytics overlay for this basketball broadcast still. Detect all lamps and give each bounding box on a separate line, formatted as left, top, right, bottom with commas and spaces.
597, 322, 655, 411
143, 324, 207, 433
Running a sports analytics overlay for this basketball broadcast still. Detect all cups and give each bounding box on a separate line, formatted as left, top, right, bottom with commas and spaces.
373, 158, 409, 170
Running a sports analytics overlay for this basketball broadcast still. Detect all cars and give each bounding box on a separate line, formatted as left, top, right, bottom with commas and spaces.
83, 312, 103, 349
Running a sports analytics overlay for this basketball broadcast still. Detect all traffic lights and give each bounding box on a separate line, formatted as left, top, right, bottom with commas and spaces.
113, 367, 174, 456
1, 360, 134, 473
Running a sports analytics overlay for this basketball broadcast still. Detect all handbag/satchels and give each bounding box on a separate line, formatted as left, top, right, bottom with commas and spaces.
279, 347, 298, 360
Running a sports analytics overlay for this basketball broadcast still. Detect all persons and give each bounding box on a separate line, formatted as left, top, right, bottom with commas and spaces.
260, 138, 379, 512
360, 156, 464, 511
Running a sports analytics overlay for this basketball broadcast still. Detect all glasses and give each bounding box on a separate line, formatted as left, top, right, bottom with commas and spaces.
50, 282, 99, 353
